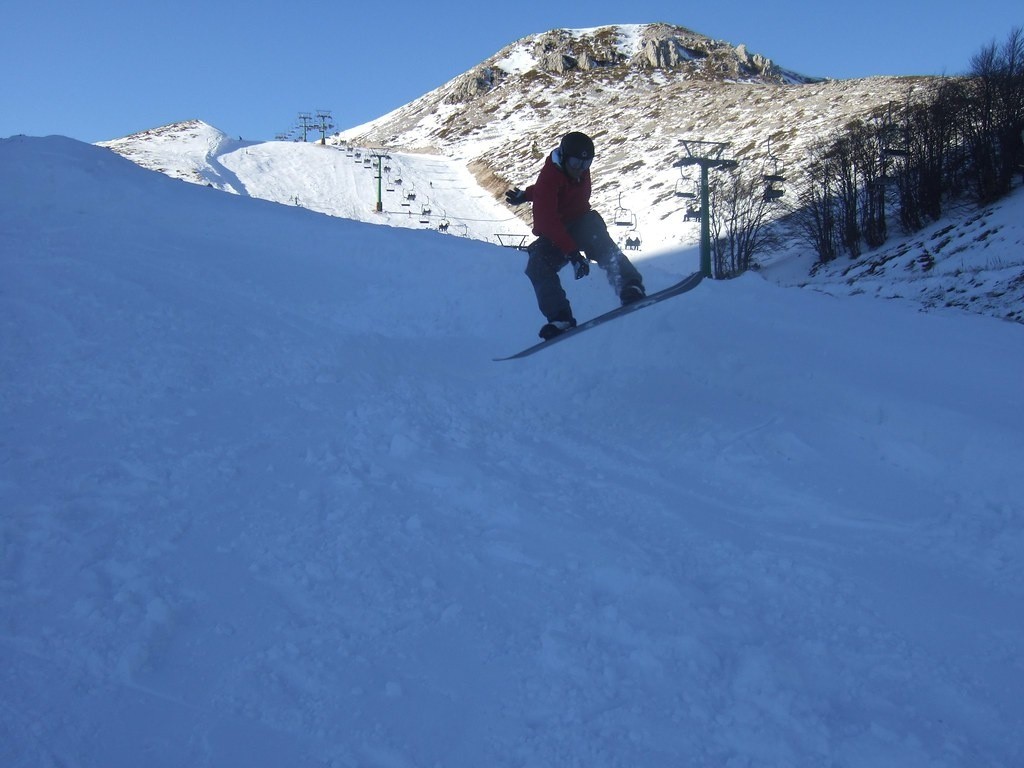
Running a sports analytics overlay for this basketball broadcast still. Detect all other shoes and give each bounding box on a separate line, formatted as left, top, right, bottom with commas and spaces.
539, 316, 577, 339
620, 284, 645, 304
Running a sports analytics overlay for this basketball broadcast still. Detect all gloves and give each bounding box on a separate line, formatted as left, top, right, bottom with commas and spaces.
565, 250, 590, 280
506, 187, 526, 206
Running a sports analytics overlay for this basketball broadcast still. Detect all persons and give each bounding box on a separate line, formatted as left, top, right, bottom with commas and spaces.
507, 132, 645, 338
695, 207, 702, 222
625, 237, 633, 249
683, 207, 694, 222
633, 237, 640, 250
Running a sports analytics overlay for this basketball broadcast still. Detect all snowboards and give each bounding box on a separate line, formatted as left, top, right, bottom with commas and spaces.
487, 268, 709, 360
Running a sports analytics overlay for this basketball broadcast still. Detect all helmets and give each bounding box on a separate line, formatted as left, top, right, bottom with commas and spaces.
560, 131, 595, 179
